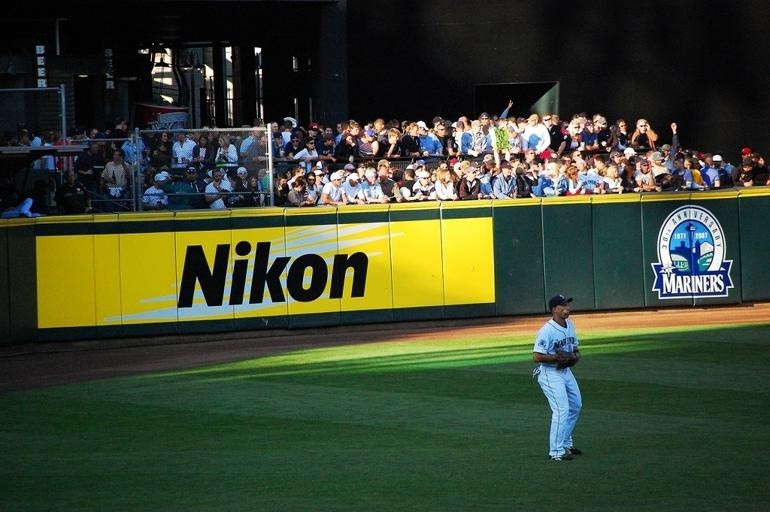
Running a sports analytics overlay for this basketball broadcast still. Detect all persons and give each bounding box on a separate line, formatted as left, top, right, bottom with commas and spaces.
0, 96, 769, 218
530, 294, 583, 461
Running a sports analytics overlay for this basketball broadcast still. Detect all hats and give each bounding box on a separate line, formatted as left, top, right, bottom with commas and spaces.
549, 295, 573, 307
313, 159, 390, 181
152, 167, 171, 183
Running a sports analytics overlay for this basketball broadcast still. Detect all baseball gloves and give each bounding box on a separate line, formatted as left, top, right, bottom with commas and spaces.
556, 349, 577, 369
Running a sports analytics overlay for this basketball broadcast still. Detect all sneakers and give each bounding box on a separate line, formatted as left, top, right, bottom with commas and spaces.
548, 447, 580, 461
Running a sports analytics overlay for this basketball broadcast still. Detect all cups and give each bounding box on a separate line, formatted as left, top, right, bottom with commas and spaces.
714, 180, 721, 188
157, 200, 161, 204
581, 142, 585, 150
